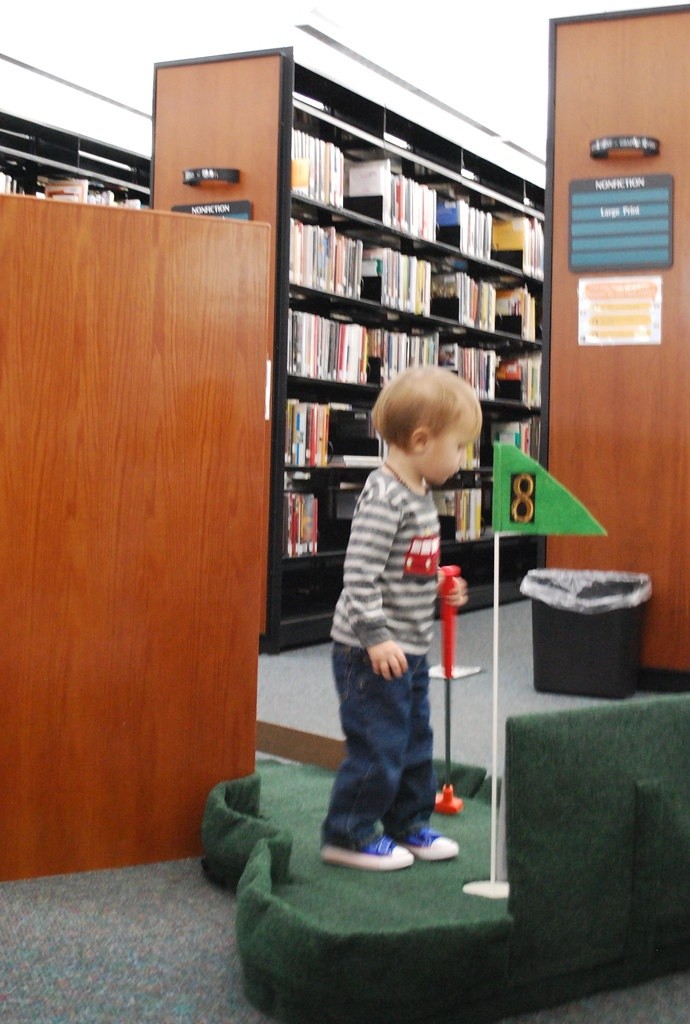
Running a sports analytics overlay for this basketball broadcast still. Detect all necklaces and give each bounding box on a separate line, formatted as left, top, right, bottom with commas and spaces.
384, 462, 429, 494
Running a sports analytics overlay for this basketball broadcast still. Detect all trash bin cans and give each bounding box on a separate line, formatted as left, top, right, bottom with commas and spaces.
528, 568, 653, 700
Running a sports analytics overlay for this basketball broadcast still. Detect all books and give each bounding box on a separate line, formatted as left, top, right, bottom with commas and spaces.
0, 171, 149, 211
287, 126, 545, 558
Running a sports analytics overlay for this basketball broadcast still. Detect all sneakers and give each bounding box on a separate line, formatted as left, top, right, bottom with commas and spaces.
382, 828, 458, 860
320, 836, 413, 870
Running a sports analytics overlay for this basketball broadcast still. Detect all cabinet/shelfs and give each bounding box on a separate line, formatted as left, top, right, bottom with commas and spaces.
537, 5, 690, 692
0, 45, 546, 656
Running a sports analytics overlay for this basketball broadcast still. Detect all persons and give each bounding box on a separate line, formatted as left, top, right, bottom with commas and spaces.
319, 366, 483, 872
